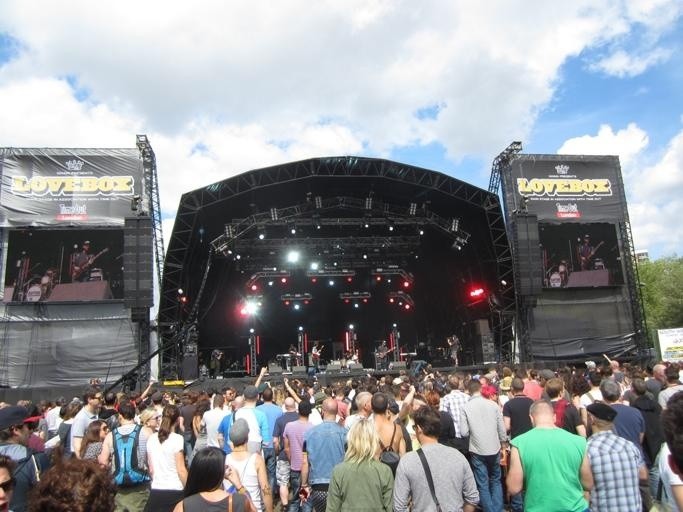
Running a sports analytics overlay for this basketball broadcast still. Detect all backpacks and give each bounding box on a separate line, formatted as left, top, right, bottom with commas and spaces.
112, 424, 151, 487
379, 422, 400, 479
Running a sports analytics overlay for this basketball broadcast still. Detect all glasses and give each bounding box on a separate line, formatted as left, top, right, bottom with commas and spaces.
103, 426, 108, 431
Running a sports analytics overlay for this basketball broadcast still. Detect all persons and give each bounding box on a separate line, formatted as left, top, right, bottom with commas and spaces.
577, 234, 596, 271
72, 240, 94, 282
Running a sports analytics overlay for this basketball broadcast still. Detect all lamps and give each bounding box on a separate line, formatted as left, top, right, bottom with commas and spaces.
252, 215, 426, 241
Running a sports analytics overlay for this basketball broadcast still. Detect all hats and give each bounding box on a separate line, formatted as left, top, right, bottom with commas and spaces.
539, 369, 554, 379
585, 361, 595, 367
500, 377, 513, 390
314, 392, 325, 402
229, 419, 248, 444
392, 377, 404, 386
0, 406, 42, 429
587, 403, 617, 421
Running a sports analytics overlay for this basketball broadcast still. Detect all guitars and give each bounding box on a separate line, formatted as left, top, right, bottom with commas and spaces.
312, 345, 324, 358
376, 347, 396, 359
72, 248, 109, 277
581, 241, 604, 266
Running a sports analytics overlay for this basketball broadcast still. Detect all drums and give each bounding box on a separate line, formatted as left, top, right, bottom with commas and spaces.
27, 286, 42, 302
550, 273, 562, 288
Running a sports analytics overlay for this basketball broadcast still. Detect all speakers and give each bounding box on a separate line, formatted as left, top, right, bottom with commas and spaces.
122, 216, 155, 309
269, 367, 282, 376
326, 366, 341, 373
389, 362, 407, 370
566, 269, 616, 286
292, 366, 307, 374
515, 213, 544, 296
48, 280, 114, 300
349, 364, 363, 372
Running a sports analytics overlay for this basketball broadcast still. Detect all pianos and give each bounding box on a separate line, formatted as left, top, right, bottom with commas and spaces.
276, 351, 302, 361
